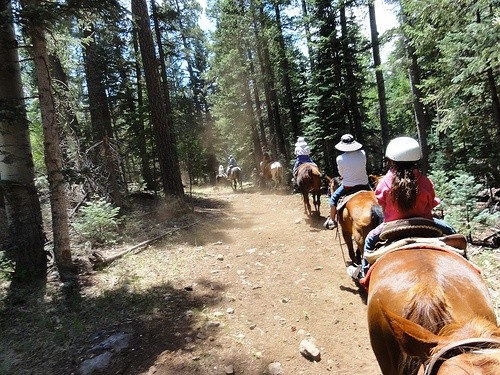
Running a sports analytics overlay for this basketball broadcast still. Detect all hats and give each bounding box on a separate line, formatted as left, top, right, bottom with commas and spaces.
335, 134, 363, 152
295, 137, 307, 146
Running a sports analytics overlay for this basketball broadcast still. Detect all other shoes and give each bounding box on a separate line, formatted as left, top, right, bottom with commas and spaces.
328, 220, 338, 226
347, 265, 364, 278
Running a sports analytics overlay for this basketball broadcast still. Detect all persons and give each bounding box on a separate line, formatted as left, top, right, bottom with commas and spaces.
323, 134, 373, 228
227, 155, 237, 176
346, 137, 457, 279
262, 146, 271, 162
291, 136, 314, 181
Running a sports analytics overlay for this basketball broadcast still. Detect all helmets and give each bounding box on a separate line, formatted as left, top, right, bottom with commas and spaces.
385, 137, 422, 161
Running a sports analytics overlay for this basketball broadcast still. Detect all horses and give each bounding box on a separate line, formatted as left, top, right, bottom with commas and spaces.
376, 298, 500, 375
216, 174, 229, 191
296, 162, 322, 224
226, 165, 245, 192
368, 173, 388, 190
323, 173, 385, 282
258, 160, 283, 193
364, 239, 498, 375
